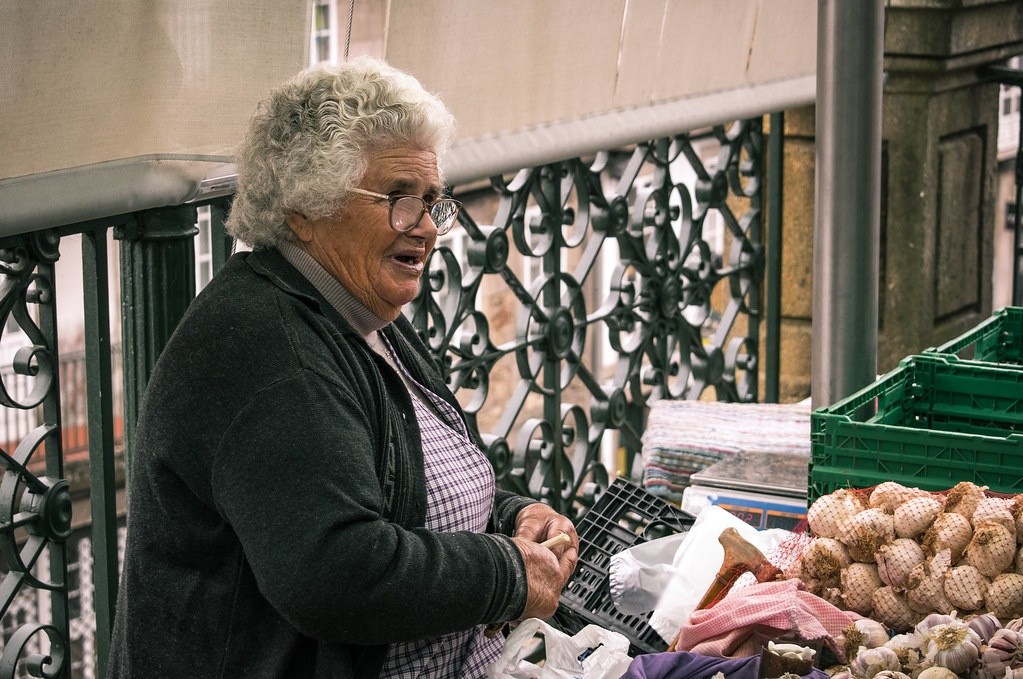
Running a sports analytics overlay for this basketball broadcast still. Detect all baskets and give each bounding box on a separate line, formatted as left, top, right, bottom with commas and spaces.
551, 476, 696, 660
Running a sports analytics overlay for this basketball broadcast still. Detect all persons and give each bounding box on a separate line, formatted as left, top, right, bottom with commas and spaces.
103, 52, 827, 679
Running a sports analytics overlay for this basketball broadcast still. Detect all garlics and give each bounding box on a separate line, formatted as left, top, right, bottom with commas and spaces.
770, 482, 1023, 679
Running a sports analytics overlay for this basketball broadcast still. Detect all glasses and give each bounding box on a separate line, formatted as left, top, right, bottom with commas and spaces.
343, 187, 463, 236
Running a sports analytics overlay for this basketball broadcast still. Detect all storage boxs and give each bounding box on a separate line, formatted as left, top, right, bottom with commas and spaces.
806, 306, 1023, 535
